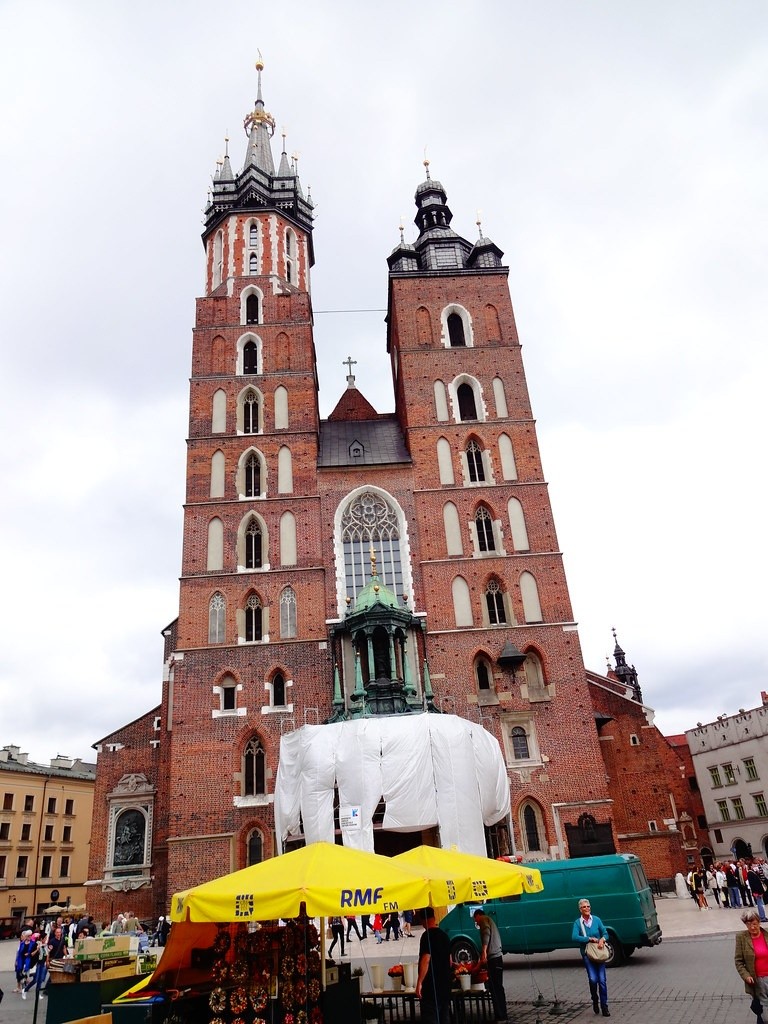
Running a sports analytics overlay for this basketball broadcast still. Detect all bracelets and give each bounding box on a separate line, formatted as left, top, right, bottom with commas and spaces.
603, 937, 606, 941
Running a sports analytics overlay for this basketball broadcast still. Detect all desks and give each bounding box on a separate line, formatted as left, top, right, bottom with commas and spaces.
101, 981, 239, 1024
361, 990, 492, 1024
46, 973, 152, 1024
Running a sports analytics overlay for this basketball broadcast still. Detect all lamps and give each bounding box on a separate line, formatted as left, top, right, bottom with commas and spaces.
8, 895, 16, 903
728, 765, 740, 782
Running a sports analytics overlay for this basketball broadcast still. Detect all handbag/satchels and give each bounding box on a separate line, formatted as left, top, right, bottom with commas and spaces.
719, 892, 726, 902
761, 881, 767, 892
584, 943, 614, 962
697, 887, 704, 894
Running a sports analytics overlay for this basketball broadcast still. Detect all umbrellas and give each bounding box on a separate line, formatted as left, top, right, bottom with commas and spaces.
172, 842, 471, 991
391, 845, 545, 911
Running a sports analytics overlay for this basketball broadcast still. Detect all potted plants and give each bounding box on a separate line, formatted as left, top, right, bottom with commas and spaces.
361, 1001, 385, 1024
353, 969, 366, 994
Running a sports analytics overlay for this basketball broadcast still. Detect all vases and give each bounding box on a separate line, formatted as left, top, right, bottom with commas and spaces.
391, 963, 418, 993
460, 974, 471, 991
371, 965, 383, 994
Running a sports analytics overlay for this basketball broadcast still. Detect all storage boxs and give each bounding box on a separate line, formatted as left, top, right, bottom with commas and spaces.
48, 935, 158, 983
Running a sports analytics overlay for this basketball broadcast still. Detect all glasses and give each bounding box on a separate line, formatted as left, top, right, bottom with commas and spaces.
28, 935, 32, 937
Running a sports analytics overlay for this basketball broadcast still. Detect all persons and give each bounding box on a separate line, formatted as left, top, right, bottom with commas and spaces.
734, 909, 768, 1024
474, 909, 508, 1020
416, 907, 452, 1024
687, 858, 768, 921
328, 910, 415, 958
572, 898, 610, 1016
12, 912, 171, 999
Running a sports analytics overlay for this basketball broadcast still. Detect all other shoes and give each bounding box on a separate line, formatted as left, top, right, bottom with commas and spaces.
724, 902, 754, 909
359, 937, 364, 941
346, 939, 352, 942
698, 906, 712, 912
592, 997, 600, 1015
718, 903, 721, 908
362, 936, 368, 938
398, 929, 403, 937
601, 1009, 611, 1017
408, 934, 415, 937
328, 950, 332, 958
393, 938, 399, 941
341, 953, 347, 957
761, 917, 768, 922
385, 938, 390, 941
377, 938, 384, 943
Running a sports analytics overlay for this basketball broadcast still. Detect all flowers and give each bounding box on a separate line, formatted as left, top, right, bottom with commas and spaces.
388, 965, 402, 978
281, 924, 323, 1024
208, 920, 272, 1024
455, 966, 472, 978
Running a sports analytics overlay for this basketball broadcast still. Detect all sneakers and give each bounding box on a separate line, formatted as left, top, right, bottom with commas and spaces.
21, 988, 27, 1000
39, 994, 44, 1000
13, 988, 22, 993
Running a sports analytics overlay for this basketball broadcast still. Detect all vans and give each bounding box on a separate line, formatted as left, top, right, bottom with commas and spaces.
438, 853, 662, 968
